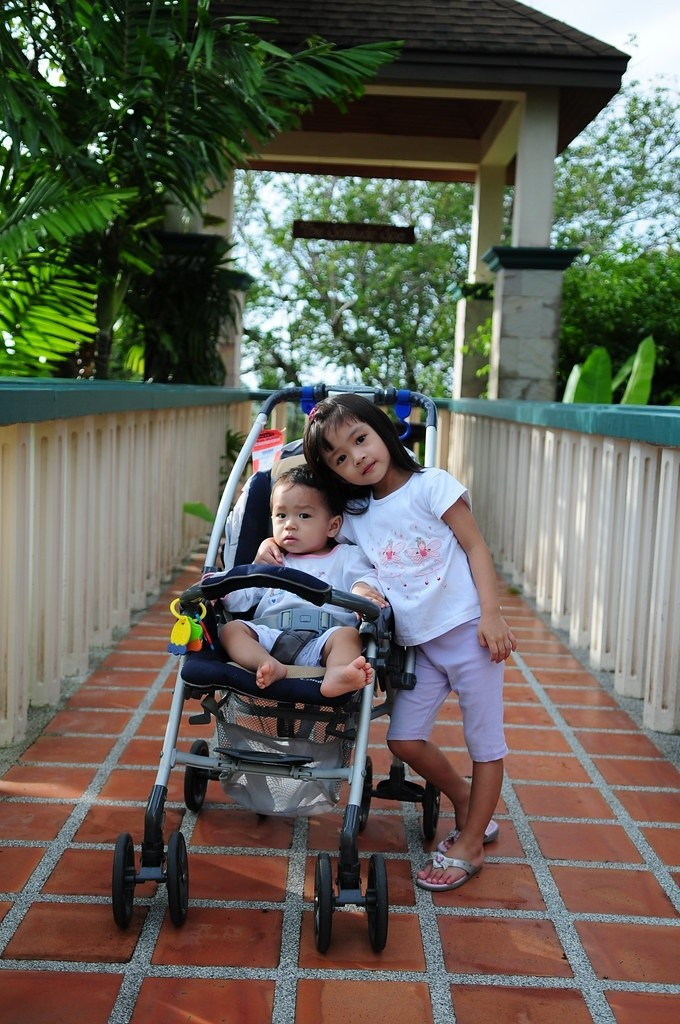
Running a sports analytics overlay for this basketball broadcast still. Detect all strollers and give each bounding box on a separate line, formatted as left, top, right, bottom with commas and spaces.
110, 383, 439, 954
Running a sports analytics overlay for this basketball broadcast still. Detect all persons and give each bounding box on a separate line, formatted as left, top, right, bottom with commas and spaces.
248, 391, 519, 893
220, 465, 390, 699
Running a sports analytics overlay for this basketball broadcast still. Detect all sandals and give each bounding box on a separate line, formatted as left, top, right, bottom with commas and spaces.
415, 818, 499, 892
